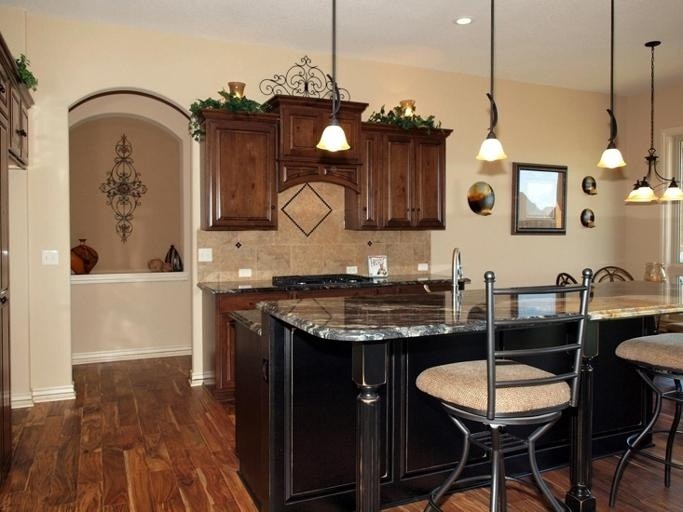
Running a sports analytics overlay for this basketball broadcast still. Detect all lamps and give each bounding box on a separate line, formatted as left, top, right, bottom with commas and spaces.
475, 0, 507, 162
596, 0, 626, 169
623, 41, 683, 202
315, 0, 350, 153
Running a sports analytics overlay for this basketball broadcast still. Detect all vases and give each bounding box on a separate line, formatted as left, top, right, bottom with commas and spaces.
71, 238, 98, 274
164, 245, 183, 271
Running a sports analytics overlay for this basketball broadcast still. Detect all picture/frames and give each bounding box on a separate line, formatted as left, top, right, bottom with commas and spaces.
367, 255, 388, 278
511, 162, 567, 235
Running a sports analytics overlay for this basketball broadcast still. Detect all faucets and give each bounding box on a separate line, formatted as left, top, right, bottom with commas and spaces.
452, 289, 463, 323
451, 248, 464, 288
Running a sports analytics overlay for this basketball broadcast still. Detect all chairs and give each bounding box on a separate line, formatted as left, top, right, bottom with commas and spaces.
415, 268, 592, 512
665, 323, 683, 332
609, 333, 682, 508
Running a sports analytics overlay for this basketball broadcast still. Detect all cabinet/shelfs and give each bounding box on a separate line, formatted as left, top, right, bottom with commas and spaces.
200, 109, 280, 230
345, 122, 453, 230
260, 94, 368, 165
0, 31, 12, 491
202, 282, 464, 401
8, 47, 34, 170
